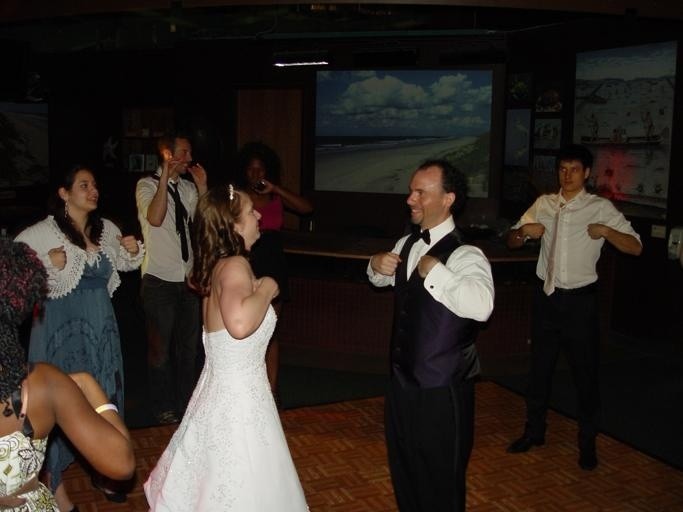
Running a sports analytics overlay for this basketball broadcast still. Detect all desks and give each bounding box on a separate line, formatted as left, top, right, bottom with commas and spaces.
282, 229, 544, 391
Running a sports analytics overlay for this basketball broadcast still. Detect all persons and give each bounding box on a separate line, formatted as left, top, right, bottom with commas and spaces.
229, 142, 315, 407
366, 159, 496, 512
143, 184, 310, 512
506, 143, 642, 471
0, 233, 136, 512
136, 133, 209, 425
13, 161, 145, 512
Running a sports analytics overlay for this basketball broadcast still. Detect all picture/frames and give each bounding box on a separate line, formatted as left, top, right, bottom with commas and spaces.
530, 81, 567, 181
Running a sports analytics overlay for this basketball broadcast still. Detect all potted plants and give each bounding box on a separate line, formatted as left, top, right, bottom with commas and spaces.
509, 79, 529, 108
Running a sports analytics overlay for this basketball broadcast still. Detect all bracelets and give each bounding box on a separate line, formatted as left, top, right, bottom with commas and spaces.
95, 403, 118, 414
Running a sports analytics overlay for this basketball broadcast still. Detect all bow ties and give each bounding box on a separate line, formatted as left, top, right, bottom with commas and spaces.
413, 228, 432, 246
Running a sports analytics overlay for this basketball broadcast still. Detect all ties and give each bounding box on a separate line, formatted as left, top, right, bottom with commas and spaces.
169, 180, 189, 263
543, 204, 562, 296
